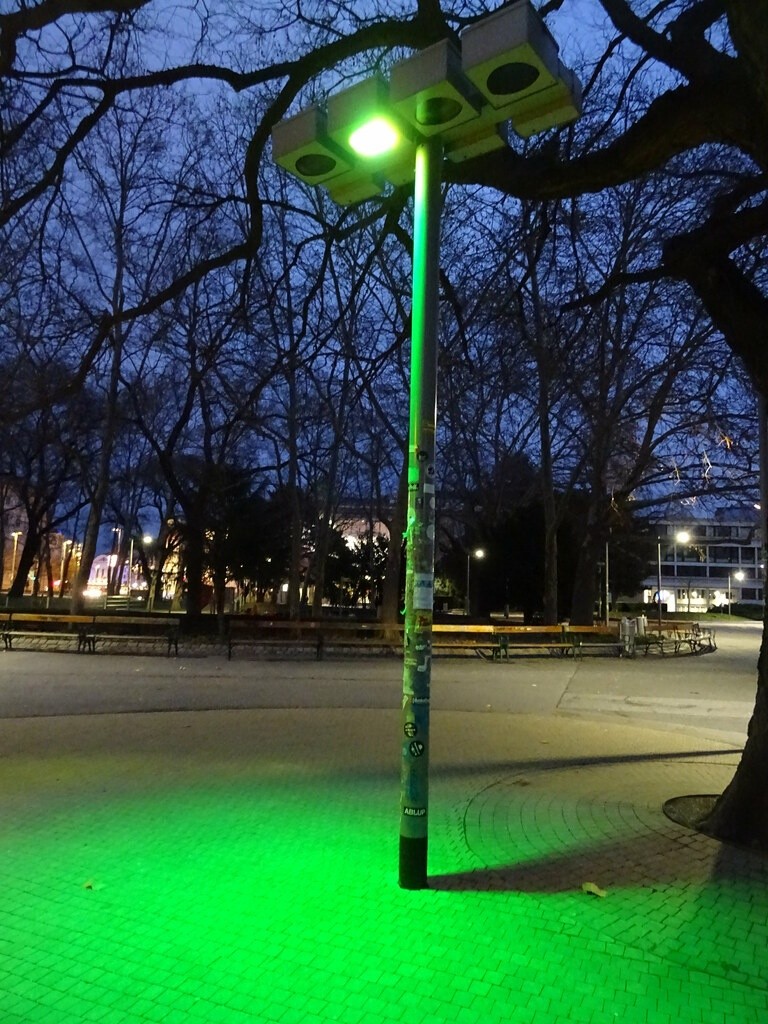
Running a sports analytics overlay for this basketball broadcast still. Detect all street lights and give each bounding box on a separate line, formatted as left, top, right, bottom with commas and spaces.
270, 0, 590, 893
60, 539, 73, 584
464, 548, 486, 622
727, 570, 747, 616
657, 531, 692, 638
111, 527, 122, 553
128, 535, 154, 597
9, 531, 24, 581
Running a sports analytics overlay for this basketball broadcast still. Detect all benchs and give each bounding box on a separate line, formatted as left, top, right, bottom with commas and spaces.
0, 611, 182, 658
432, 624, 631, 664
223, 621, 405, 662
645, 622, 718, 656
